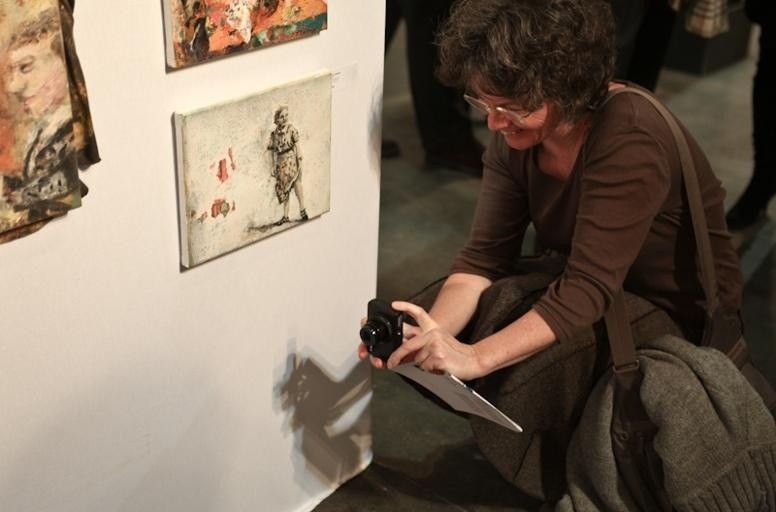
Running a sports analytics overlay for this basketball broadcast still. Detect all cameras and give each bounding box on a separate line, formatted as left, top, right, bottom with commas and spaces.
360, 299, 403, 363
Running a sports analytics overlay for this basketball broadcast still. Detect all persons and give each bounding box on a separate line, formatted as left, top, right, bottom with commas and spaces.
4, 6, 73, 184
267, 105, 309, 226
382, 0, 486, 179
359, 1, 750, 506
726, 1, 776, 233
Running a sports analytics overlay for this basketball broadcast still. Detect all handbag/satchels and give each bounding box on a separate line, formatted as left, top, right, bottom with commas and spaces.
608, 299, 775, 512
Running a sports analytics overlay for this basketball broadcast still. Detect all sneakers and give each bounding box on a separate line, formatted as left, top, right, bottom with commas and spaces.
381, 131, 487, 175
726, 188, 771, 230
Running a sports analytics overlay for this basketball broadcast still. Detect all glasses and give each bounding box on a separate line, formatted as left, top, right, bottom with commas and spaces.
461, 87, 547, 128
